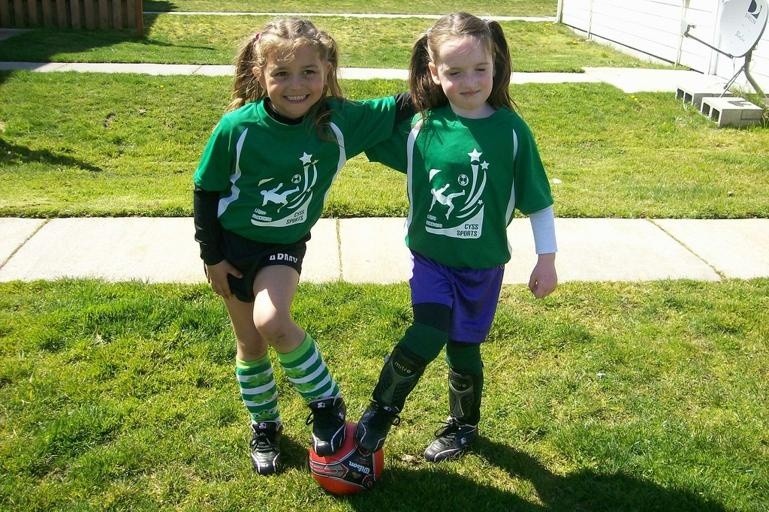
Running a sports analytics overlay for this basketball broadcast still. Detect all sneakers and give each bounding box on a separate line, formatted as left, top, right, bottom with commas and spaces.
355, 398, 397, 454
423, 418, 478, 462
250, 417, 283, 475
308, 396, 346, 457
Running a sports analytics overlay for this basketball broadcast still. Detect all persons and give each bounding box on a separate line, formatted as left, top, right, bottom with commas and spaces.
354, 12, 557, 462
193, 18, 444, 475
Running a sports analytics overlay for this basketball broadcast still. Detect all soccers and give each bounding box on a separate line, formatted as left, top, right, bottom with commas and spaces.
309, 423, 384, 496
